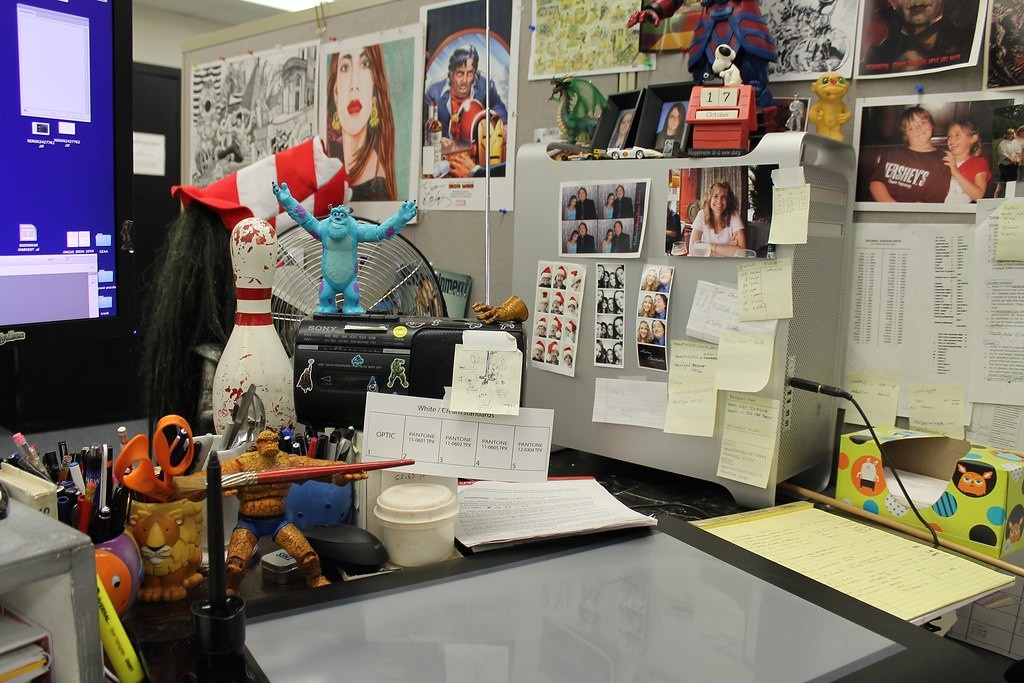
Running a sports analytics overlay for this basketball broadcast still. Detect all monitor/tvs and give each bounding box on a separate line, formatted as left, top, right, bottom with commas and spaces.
0, 0, 141, 350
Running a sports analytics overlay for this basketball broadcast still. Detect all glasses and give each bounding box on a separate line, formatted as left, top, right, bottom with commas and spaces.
905, 119, 930, 131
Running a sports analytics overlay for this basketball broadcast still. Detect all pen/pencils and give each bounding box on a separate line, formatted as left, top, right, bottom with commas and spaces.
118, 426, 135, 498
6, 432, 128, 545
277, 424, 355, 463
158, 428, 213, 482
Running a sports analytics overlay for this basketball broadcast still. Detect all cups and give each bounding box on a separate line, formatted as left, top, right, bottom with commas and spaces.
127, 499, 203, 601
187, 434, 244, 548
94, 530, 143, 618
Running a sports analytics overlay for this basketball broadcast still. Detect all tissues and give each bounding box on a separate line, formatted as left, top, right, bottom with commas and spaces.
835, 427, 1024, 561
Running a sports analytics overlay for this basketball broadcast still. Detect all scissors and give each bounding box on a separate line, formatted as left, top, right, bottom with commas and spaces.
115, 415, 195, 504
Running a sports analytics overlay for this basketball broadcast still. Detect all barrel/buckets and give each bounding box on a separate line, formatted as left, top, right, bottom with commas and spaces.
375, 483, 459, 568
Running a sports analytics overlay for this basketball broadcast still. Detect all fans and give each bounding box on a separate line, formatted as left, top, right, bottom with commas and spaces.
269, 212, 447, 360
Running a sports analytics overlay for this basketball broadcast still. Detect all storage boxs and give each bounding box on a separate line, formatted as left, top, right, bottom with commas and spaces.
835, 426, 1023, 556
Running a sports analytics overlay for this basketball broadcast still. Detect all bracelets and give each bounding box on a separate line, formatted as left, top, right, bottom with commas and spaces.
714, 244, 718, 253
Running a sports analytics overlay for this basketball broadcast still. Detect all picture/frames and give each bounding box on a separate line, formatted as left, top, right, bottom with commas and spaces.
634, 80, 700, 151
589, 87, 646, 157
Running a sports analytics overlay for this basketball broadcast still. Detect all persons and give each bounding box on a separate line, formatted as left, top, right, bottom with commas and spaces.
666, 181, 748, 257
271, 182, 419, 315
565, 185, 635, 253
637, 266, 673, 346
596, 264, 625, 365
940, 119, 993, 206
537, 292, 580, 318
626, 0, 785, 131
866, 109, 952, 204
187, 429, 369, 596
532, 340, 574, 368
610, 111, 634, 149
534, 316, 576, 343
994, 126, 1024, 198
539, 265, 581, 291
656, 103, 686, 148
471, 296, 529, 324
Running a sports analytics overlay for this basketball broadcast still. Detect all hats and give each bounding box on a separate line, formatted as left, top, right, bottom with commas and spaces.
532, 266, 581, 363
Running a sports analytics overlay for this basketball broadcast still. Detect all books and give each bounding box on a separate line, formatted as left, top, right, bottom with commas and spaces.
0, 600, 49, 683
0, 462, 60, 521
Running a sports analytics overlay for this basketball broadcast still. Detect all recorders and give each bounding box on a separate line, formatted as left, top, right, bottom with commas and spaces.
293, 312, 527, 432
